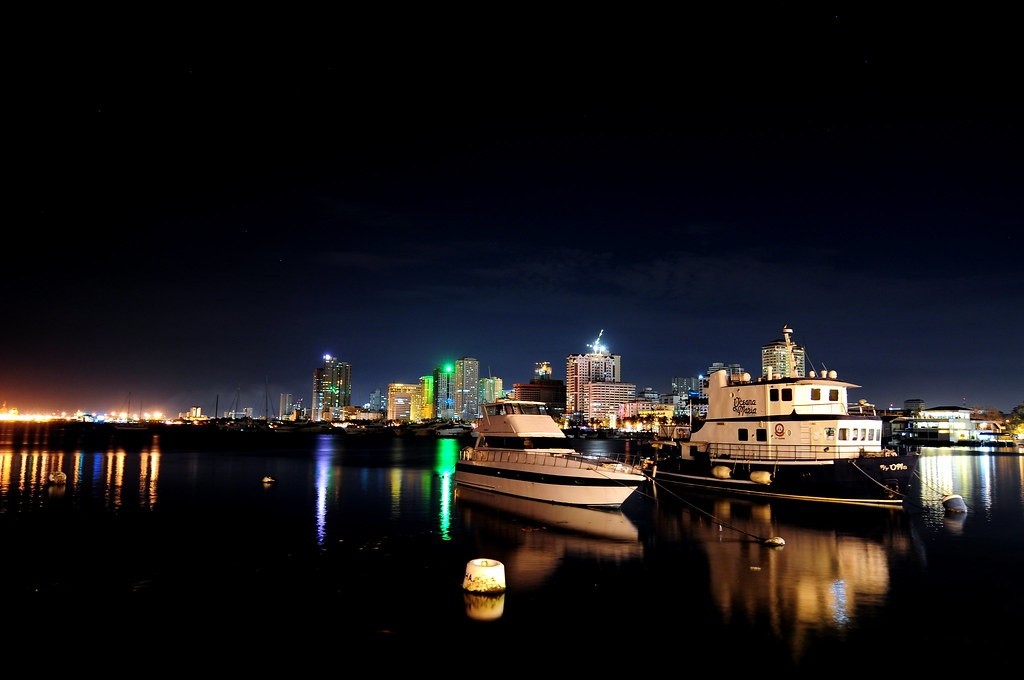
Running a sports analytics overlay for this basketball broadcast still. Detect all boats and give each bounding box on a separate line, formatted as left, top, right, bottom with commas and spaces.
396, 415, 473, 436
641, 323, 923, 508
643, 478, 909, 648
454, 396, 648, 508
454, 486, 643, 559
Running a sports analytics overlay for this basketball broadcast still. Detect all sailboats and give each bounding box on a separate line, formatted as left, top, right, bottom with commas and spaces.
114, 391, 148, 430
206, 374, 325, 434
